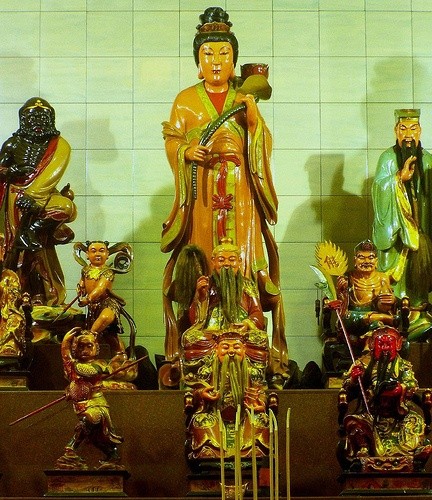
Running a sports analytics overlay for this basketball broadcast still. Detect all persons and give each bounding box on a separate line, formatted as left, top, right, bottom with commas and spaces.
181, 237, 269, 349
161, 6, 282, 310
192, 332, 277, 459
0, 96, 76, 250
369, 105, 432, 342
74, 239, 122, 341
326, 239, 398, 329
61, 326, 126, 462
343, 325, 431, 474
